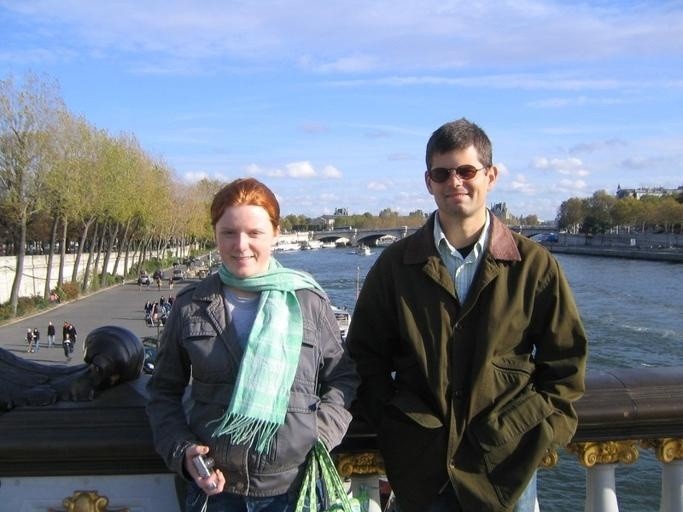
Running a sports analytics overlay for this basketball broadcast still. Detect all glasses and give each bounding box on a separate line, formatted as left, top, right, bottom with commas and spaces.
428, 164, 485, 184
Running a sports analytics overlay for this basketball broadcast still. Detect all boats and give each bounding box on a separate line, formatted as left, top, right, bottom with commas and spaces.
356, 241, 372, 257
300, 240, 311, 251
320, 241, 337, 248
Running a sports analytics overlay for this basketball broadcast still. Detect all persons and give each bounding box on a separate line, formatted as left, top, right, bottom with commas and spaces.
46, 321, 55, 348
60, 321, 77, 360
32, 328, 40, 352
23, 328, 34, 353
141, 177, 361, 512
137, 274, 176, 328
343, 116, 588, 511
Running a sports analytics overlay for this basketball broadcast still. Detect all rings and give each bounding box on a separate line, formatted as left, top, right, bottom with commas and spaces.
208, 482, 216, 490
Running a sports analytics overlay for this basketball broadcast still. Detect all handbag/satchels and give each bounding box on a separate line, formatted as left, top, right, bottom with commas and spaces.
294, 440, 370, 512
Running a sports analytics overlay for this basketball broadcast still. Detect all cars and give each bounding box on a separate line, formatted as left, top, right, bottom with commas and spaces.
172, 268, 185, 280
139, 269, 164, 285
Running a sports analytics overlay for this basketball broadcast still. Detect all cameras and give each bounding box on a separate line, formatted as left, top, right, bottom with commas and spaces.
192, 454, 216, 479
